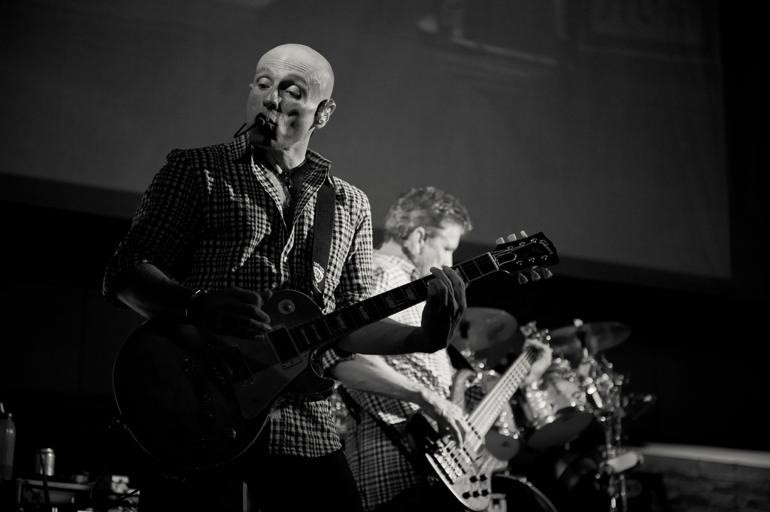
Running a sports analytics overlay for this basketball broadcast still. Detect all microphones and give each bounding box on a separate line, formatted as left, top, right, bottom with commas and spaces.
232, 112, 271, 138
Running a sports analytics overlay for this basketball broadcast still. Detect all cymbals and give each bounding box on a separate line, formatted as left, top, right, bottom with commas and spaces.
551, 320, 628, 356
446, 310, 517, 352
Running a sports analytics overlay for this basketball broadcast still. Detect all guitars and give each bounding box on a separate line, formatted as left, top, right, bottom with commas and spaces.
407, 328, 553, 511
111, 228, 558, 476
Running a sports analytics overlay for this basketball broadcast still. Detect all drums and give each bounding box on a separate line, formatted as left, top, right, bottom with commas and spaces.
519, 359, 593, 449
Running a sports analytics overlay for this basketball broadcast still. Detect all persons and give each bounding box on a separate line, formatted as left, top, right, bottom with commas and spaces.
322, 186, 553, 512
101, 43, 469, 512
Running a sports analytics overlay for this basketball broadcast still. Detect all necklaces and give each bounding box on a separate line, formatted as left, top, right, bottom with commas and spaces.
260, 155, 308, 202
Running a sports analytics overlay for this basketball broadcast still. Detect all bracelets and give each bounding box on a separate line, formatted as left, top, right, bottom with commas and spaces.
185, 288, 208, 318
